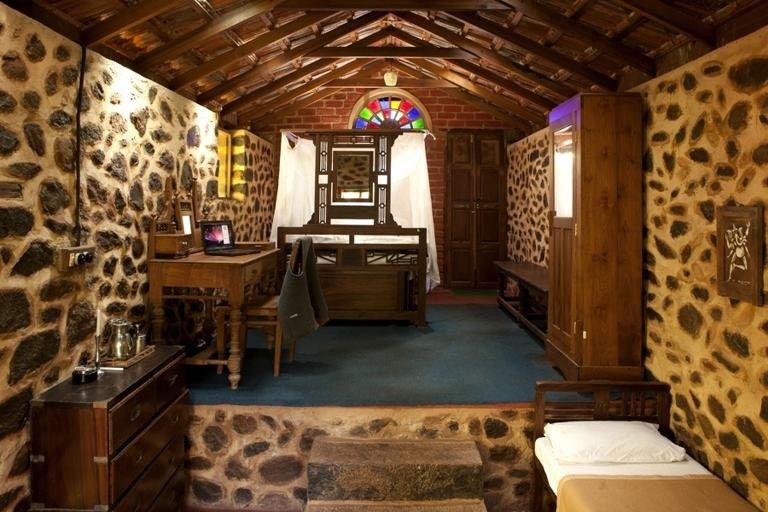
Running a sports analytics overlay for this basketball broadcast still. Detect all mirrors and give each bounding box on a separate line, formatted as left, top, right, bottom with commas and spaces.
174, 198, 196, 247
549, 111, 577, 230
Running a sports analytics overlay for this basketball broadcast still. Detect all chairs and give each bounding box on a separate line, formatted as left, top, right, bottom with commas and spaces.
214, 235, 330, 377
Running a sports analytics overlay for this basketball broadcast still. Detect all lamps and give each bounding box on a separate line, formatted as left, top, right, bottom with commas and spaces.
383, 18, 403, 87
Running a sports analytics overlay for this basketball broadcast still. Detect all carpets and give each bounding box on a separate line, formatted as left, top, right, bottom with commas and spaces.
187, 303, 656, 408
452, 288, 501, 296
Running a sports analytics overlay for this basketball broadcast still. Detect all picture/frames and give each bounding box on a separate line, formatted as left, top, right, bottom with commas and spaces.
716, 204, 763, 308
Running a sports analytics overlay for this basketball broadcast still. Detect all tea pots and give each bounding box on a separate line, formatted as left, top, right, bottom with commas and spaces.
107, 316, 134, 360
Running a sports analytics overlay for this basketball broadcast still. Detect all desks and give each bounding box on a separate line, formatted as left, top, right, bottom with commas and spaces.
147, 247, 283, 390
494, 258, 549, 344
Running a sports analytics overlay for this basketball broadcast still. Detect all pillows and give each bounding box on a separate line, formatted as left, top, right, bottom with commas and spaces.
543, 420, 686, 465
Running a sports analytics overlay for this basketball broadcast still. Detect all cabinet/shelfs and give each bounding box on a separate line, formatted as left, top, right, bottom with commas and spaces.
547, 90, 644, 395
28, 344, 195, 512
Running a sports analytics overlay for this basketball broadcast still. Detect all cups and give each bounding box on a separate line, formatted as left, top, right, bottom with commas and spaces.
132, 334, 146, 355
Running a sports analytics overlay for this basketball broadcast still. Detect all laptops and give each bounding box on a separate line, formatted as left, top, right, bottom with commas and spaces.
200, 221, 261, 256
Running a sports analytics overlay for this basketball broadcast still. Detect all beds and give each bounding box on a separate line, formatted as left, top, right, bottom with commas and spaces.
277, 128, 428, 328
531, 379, 762, 512
